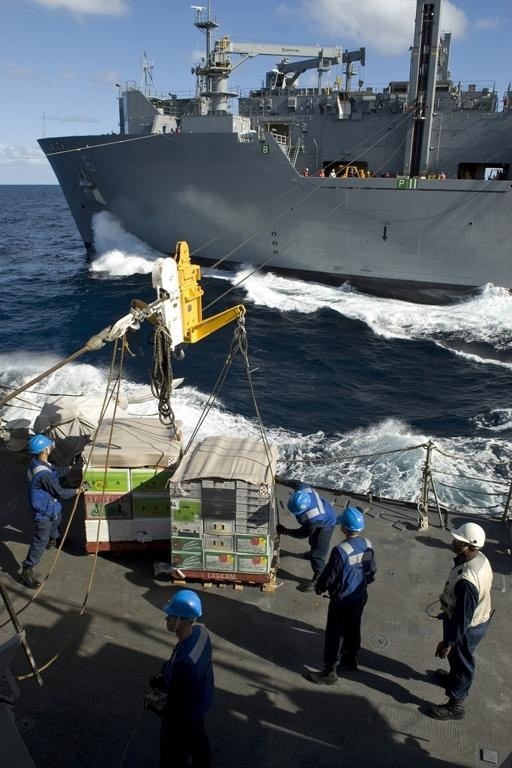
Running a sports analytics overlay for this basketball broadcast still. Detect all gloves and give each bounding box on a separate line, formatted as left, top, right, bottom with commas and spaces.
81, 481, 90, 492
275, 475, 280, 484
149, 673, 163, 689
276, 524, 287, 535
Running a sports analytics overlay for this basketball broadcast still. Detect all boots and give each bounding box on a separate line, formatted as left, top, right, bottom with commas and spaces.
435, 668, 448, 688
428, 700, 465, 720
47, 538, 57, 550
309, 664, 335, 683
20, 568, 40, 588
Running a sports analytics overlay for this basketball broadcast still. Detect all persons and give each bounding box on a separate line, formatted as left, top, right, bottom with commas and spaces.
429, 522, 494, 721
274, 483, 337, 593
306, 507, 377, 684
149, 590, 215, 768
20, 435, 91, 590
302, 166, 448, 181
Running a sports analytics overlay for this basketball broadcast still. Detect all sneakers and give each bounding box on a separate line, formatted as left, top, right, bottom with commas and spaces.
303, 580, 317, 592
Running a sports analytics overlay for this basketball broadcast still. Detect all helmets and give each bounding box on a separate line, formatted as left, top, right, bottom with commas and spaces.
338, 508, 365, 532
287, 491, 311, 516
451, 522, 486, 548
164, 591, 202, 618
28, 434, 53, 454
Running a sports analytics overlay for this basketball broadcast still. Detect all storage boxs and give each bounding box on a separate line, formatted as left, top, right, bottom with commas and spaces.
83, 457, 280, 575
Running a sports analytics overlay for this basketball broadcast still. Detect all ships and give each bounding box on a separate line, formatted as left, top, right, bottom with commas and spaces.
37, 0, 511, 310
